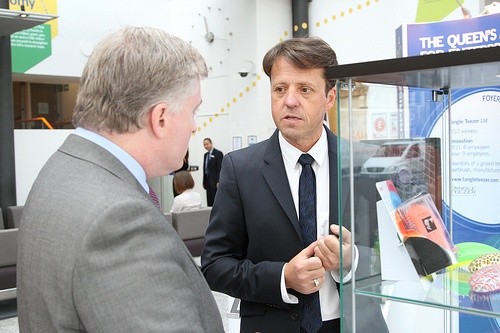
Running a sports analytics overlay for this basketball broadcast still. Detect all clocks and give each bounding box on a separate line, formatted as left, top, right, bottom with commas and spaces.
189, 6, 233, 71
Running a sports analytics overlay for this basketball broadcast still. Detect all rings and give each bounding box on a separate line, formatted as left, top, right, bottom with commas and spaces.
313, 279, 320, 287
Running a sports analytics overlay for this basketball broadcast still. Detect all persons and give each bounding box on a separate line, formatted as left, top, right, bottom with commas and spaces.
201, 39, 382, 333
15, 27, 224, 333
171, 170, 203, 212
202, 138, 223, 207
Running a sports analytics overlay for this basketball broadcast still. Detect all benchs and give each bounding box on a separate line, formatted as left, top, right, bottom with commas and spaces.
0, 227, 19, 301
171, 207, 213, 256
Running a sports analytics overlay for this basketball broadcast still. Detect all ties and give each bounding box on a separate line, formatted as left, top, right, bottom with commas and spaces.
207, 153, 210, 176
295, 153, 322, 333
149, 186, 160, 208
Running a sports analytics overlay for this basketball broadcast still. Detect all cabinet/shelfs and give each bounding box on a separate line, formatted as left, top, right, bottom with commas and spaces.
324, 45, 500, 333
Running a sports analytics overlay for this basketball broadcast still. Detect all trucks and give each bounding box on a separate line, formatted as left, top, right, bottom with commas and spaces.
359, 136, 442, 180
